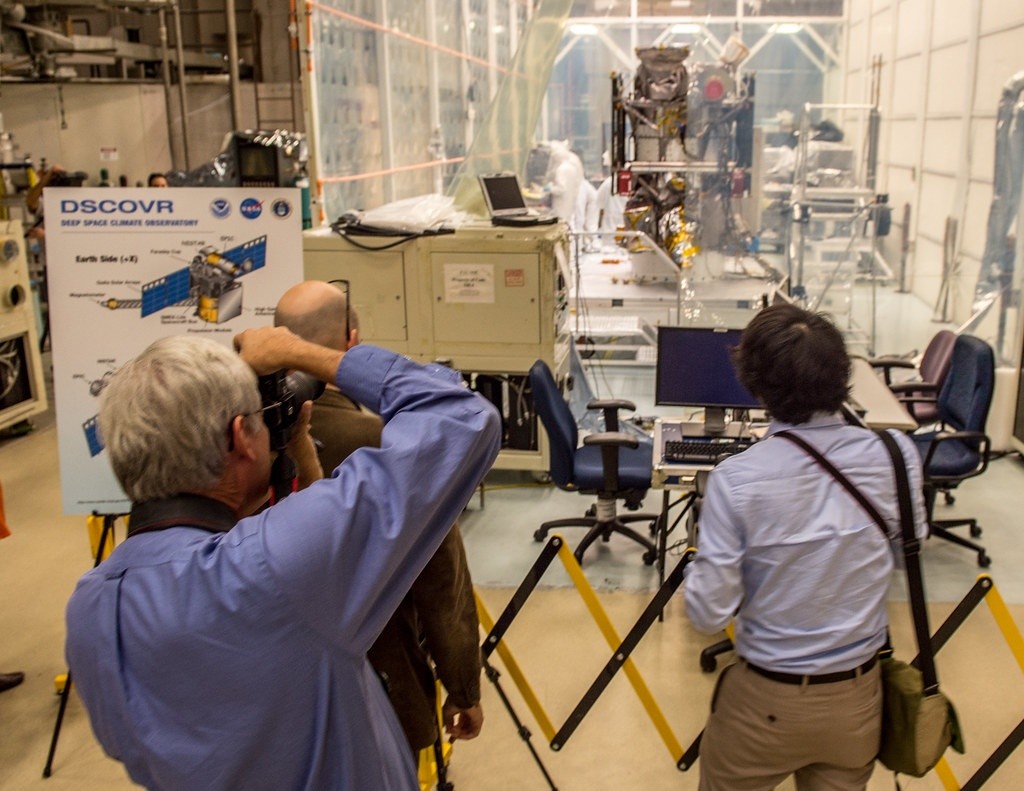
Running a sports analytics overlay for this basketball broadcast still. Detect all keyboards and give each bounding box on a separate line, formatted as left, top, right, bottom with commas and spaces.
664, 441, 754, 464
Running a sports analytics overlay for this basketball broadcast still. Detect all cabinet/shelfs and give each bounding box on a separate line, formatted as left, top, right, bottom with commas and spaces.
302, 221, 570, 483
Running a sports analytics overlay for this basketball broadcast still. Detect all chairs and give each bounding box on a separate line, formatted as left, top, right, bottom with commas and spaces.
903, 334, 997, 567
867, 329, 959, 426
529, 359, 662, 567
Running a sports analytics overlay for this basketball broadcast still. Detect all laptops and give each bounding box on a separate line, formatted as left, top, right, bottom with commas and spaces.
479, 172, 558, 227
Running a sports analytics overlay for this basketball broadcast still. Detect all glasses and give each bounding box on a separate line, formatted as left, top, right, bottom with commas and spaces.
227, 396, 282, 452
328, 279, 351, 341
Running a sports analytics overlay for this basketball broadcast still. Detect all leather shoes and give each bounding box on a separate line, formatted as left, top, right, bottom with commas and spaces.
0, 672, 24, 692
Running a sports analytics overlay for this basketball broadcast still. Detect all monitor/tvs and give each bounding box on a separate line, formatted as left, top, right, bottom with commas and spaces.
655, 326, 767, 439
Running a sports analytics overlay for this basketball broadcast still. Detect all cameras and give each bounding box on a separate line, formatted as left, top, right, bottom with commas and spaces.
258, 368, 327, 452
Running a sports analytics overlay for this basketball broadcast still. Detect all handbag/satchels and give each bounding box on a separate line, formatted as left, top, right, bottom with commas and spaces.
875, 656, 965, 777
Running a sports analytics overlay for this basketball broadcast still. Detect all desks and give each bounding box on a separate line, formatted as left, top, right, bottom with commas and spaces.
841, 356, 919, 435
651, 415, 773, 622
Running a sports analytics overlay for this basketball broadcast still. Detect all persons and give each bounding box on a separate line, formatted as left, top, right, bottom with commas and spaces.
275, 282, 482, 779
765, 111, 798, 148
684, 304, 928, 791
64, 324, 500, 791
24, 165, 172, 352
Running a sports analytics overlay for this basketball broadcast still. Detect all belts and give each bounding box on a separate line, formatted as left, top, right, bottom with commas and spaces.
738, 655, 878, 684
